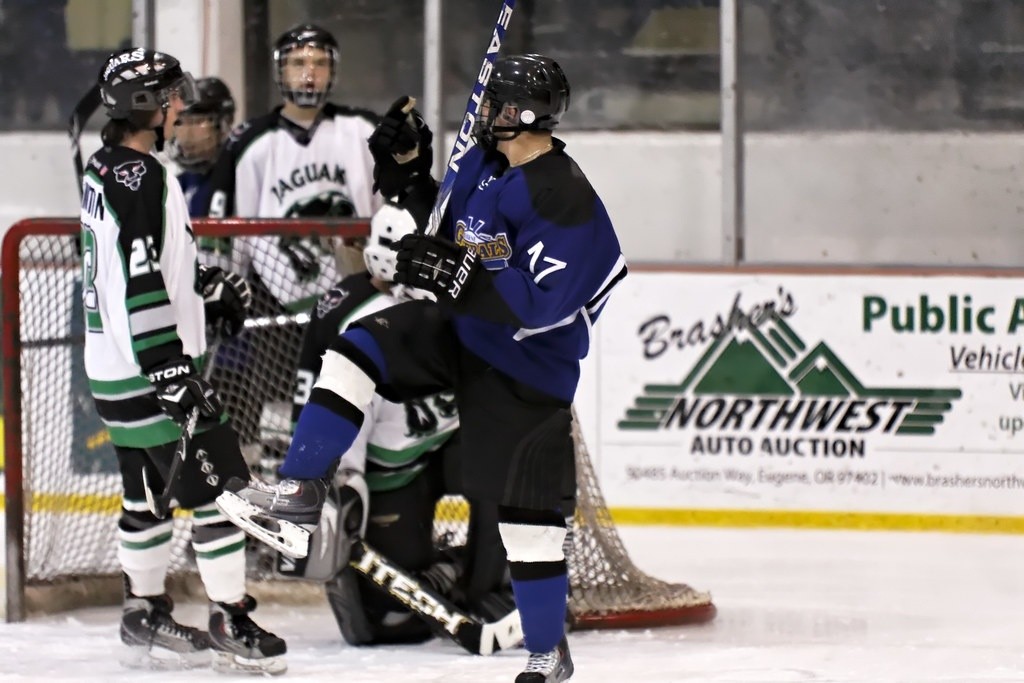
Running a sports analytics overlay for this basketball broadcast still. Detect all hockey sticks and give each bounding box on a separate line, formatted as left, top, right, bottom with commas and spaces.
69, 84, 102, 200
347, 539, 523, 656
244, 309, 315, 330
424, 0, 516, 237
142, 318, 221, 518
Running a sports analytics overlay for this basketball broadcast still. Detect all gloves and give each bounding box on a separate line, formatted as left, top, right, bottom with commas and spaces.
194, 266, 244, 338
146, 355, 224, 427
367, 96, 433, 205
393, 234, 482, 304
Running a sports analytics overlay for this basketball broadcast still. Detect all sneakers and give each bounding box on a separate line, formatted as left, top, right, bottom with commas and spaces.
207, 594, 288, 674
215, 478, 331, 559
116, 570, 212, 670
515, 634, 574, 683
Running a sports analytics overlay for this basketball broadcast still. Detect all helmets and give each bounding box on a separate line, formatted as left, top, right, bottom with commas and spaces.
364, 207, 422, 292
470, 53, 571, 151
274, 23, 339, 50
177, 76, 235, 115
98, 49, 185, 119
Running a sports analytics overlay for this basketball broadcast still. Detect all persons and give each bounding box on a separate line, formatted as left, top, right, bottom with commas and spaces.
303, 196, 510, 646
225, 22, 383, 441
77, 48, 287, 677
216, 52, 629, 683
173, 77, 265, 437
